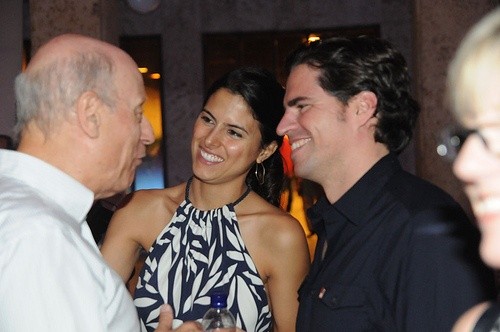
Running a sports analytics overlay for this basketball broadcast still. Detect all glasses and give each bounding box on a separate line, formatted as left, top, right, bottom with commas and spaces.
437, 126, 500, 163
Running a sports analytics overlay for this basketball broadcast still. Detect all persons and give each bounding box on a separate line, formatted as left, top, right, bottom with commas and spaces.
445, 4, 499, 332
276, 33, 495, 332
98, 68, 312, 332
0, 32, 158, 332
